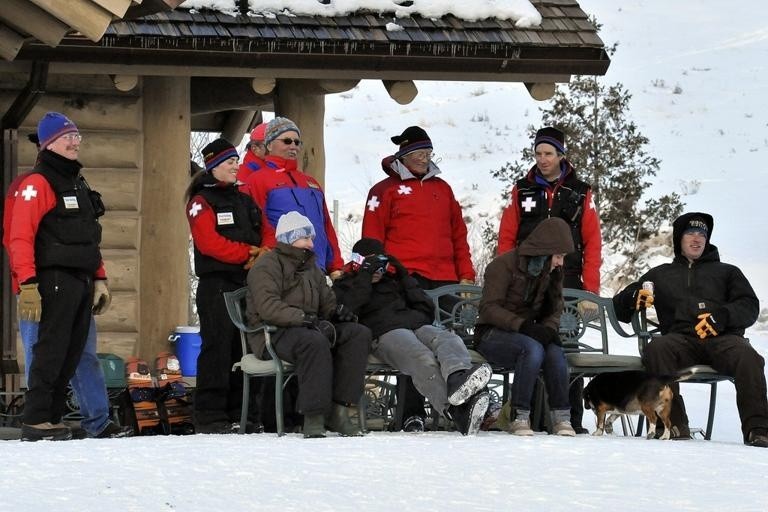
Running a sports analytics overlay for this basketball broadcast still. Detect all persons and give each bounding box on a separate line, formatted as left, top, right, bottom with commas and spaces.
612, 212, 768, 448
234, 123, 271, 186
361, 125, 476, 432
2, 112, 111, 440
496, 128, 602, 435
247, 212, 373, 438
186, 137, 269, 426
3, 132, 135, 437
332, 237, 493, 437
239, 116, 344, 285
478, 217, 579, 438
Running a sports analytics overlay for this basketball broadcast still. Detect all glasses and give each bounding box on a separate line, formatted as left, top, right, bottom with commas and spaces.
60, 134, 83, 142
353, 252, 389, 274
274, 138, 301, 146
536, 152, 562, 156
415, 152, 435, 159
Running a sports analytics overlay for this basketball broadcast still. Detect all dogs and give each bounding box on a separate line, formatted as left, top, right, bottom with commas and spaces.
582, 369, 673, 440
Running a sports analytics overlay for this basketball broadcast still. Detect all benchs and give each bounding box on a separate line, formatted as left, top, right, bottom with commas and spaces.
223, 284, 662, 437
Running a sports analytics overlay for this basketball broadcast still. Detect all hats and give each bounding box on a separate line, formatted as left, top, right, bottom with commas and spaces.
201, 138, 240, 172
681, 217, 708, 237
354, 239, 384, 255
250, 123, 267, 143
391, 126, 433, 158
534, 127, 565, 153
37, 112, 78, 152
264, 116, 300, 146
274, 211, 316, 245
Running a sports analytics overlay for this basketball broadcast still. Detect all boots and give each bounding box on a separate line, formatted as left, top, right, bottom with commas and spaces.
549, 408, 576, 436
327, 400, 364, 435
447, 388, 490, 437
507, 407, 534, 436
447, 362, 492, 406
302, 407, 327, 438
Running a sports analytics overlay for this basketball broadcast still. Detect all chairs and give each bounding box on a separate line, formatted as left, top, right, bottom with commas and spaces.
631, 306, 749, 441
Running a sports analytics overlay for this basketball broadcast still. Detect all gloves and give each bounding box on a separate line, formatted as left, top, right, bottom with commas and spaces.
385, 254, 408, 282
329, 305, 358, 322
90, 278, 111, 317
460, 279, 475, 300
620, 287, 654, 311
518, 318, 540, 340
331, 270, 345, 284
696, 309, 727, 339
19, 284, 42, 323
539, 320, 558, 345
577, 292, 598, 319
359, 256, 384, 275
301, 315, 337, 349
243, 247, 270, 270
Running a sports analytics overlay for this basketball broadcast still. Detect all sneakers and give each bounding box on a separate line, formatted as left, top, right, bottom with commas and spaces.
654, 423, 691, 440
53, 422, 88, 440
572, 421, 589, 435
194, 418, 241, 433
235, 418, 265, 433
22, 422, 70, 442
744, 427, 768, 447
401, 412, 425, 431
263, 412, 305, 433
89, 423, 133, 438
